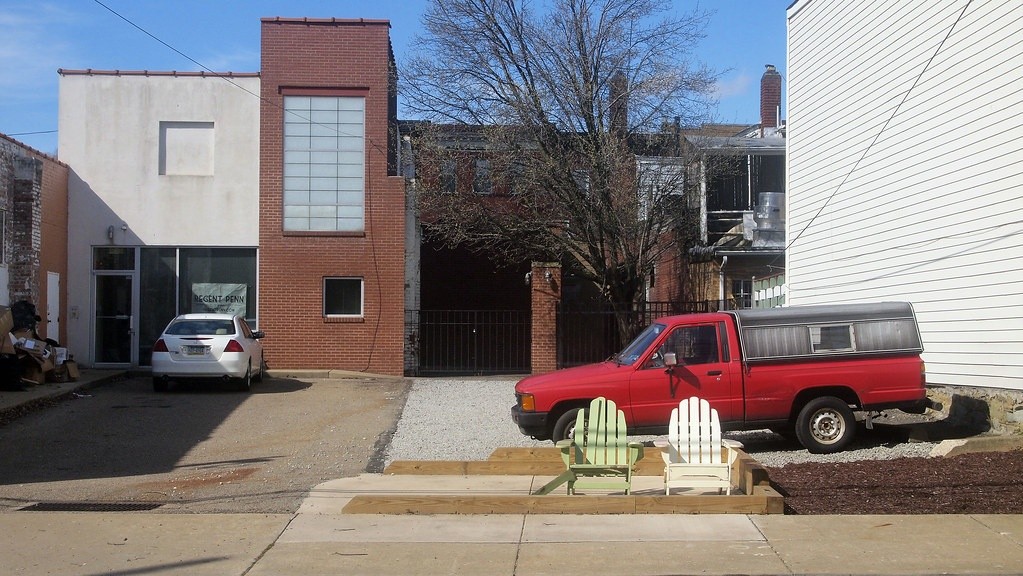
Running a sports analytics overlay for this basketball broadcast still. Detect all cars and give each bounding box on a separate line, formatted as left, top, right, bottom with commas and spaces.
150, 313, 265, 391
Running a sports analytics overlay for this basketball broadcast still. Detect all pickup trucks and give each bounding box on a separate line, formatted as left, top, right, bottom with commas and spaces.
509, 301, 928, 456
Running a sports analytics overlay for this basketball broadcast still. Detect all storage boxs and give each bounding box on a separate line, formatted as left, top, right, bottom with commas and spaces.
54, 362, 81, 383
53, 347, 66, 365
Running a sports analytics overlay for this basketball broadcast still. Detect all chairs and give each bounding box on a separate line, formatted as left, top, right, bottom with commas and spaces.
179, 328, 192, 335
652, 395, 744, 496
216, 328, 228, 335
554, 395, 644, 496
